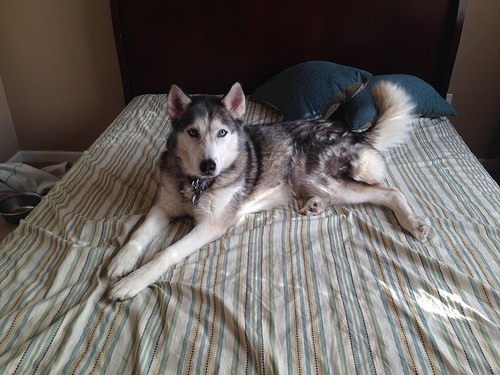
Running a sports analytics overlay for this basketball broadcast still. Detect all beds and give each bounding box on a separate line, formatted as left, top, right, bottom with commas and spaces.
0, 0, 499, 375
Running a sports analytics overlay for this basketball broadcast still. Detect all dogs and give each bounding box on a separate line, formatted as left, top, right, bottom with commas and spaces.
104, 81, 430, 301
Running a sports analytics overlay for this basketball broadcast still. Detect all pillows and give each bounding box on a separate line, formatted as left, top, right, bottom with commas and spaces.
251, 59, 371, 130
335, 72, 455, 136
